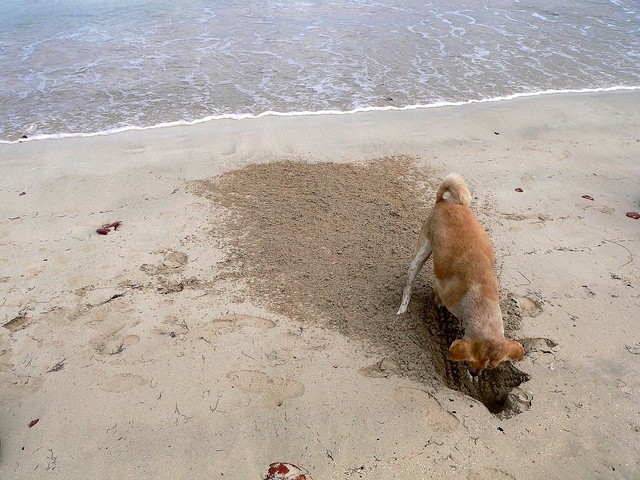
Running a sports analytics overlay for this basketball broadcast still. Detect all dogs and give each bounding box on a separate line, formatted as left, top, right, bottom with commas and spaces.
397, 173, 525, 378
